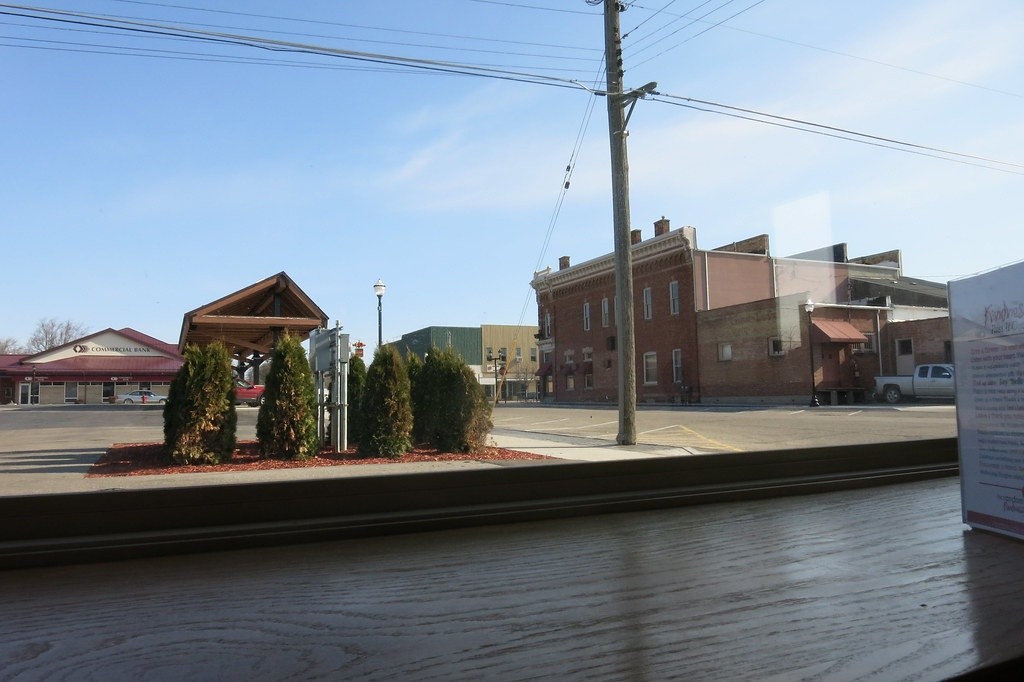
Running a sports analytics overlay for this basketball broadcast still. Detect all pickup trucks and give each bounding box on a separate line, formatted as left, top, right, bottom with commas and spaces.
872, 363, 956, 405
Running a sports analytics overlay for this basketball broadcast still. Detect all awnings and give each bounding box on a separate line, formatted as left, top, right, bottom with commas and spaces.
810, 322, 868, 343
535, 362, 553, 376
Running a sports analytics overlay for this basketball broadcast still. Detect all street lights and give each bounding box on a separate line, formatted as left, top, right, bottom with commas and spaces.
373, 278, 385, 350
486, 347, 506, 406
803, 298, 819, 407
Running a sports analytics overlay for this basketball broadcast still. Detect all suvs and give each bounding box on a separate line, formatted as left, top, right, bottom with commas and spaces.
229, 377, 267, 407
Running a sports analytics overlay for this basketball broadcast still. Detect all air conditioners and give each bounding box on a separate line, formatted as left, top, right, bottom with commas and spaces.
515, 357, 522, 363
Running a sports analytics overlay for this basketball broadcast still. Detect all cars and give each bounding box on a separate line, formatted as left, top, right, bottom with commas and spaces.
115, 390, 170, 405
